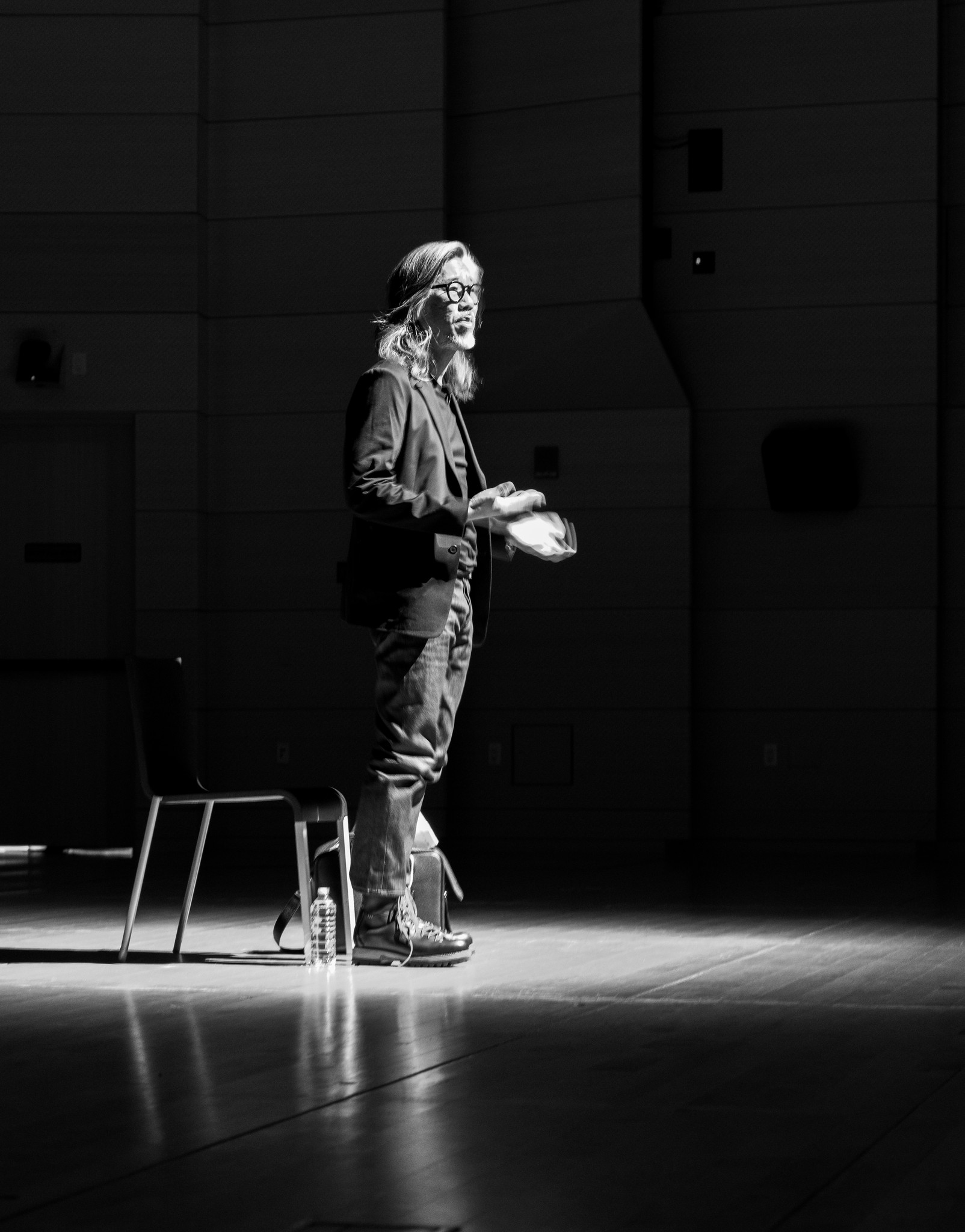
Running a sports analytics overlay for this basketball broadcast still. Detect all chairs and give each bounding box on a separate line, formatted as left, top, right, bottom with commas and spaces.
115, 651, 356, 968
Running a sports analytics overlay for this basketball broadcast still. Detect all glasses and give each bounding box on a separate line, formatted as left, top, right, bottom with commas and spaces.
431, 280, 485, 305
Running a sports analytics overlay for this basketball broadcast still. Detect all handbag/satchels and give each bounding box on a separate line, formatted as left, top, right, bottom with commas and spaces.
271, 822, 465, 954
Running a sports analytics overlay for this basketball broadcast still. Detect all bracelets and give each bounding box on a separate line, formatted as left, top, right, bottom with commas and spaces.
505, 537, 515, 552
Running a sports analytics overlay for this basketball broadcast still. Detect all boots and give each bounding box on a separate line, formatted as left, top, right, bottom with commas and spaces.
350, 891, 475, 967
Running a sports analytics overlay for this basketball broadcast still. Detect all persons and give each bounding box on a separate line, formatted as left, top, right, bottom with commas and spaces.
336, 234, 572, 968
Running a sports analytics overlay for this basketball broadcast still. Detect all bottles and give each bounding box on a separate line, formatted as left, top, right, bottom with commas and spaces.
310, 885, 337, 970
